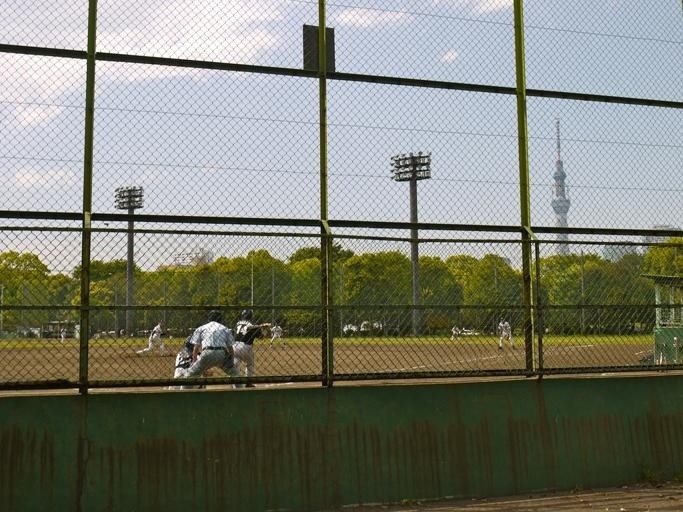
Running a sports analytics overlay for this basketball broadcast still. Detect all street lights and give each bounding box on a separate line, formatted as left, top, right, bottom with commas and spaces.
390, 150, 430, 339
114, 185, 143, 336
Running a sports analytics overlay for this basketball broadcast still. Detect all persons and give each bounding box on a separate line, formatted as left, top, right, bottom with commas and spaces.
269, 321, 285, 348
60, 324, 67, 344
133, 319, 167, 358
449, 324, 461, 341
167, 334, 214, 389
498, 317, 514, 351
178, 308, 244, 389
231, 308, 271, 388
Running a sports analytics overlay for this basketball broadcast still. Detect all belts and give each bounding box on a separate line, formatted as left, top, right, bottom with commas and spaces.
202, 346, 225, 351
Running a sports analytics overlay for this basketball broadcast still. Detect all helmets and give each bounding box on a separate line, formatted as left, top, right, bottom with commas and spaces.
240, 309, 253, 321
207, 309, 223, 322
